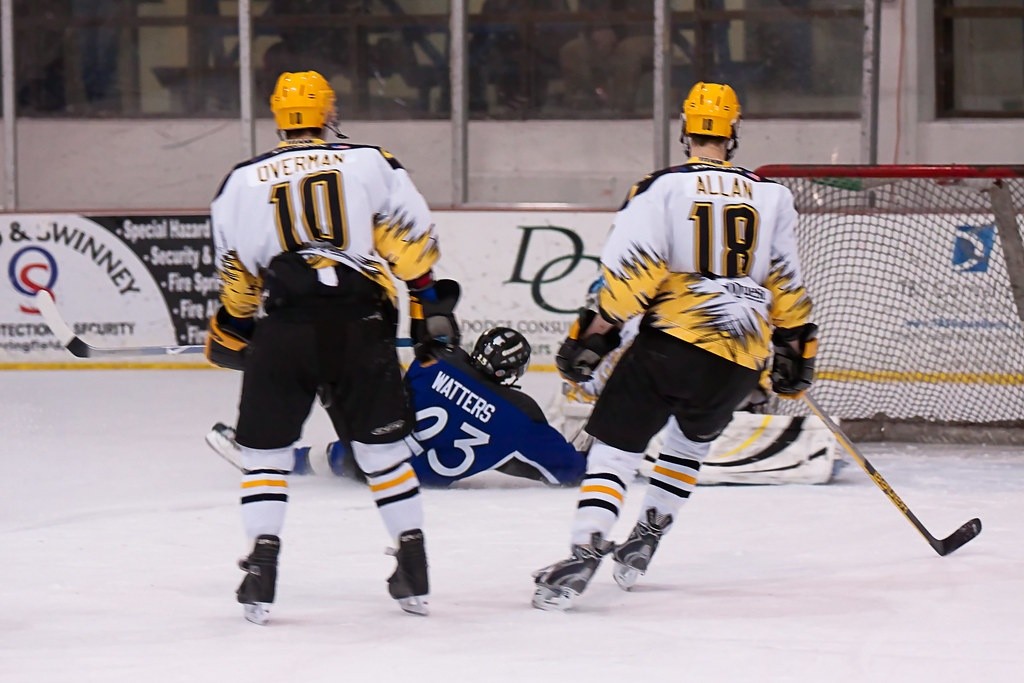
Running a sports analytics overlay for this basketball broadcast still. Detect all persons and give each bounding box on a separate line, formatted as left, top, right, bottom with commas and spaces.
205, 70, 456, 626
532, 80, 819, 611
205, 278, 586, 487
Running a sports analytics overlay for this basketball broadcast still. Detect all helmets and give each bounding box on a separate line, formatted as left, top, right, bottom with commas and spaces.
268, 69, 337, 130
680, 81, 742, 139
470, 326, 532, 387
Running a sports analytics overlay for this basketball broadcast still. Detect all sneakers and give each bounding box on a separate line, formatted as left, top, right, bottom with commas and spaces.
529, 531, 616, 611
611, 506, 675, 591
384, 528, 433, 615
205, 421, 251, 477
236, 534, 281, 626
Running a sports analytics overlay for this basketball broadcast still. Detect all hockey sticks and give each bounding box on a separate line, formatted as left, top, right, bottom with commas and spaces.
800, 387, 982, 560
35, 287, 207, 360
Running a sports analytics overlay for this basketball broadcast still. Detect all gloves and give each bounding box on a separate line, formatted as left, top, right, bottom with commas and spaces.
408, 280, 462, 363
554, 306, 621, 389
768, 322, 819, 395
